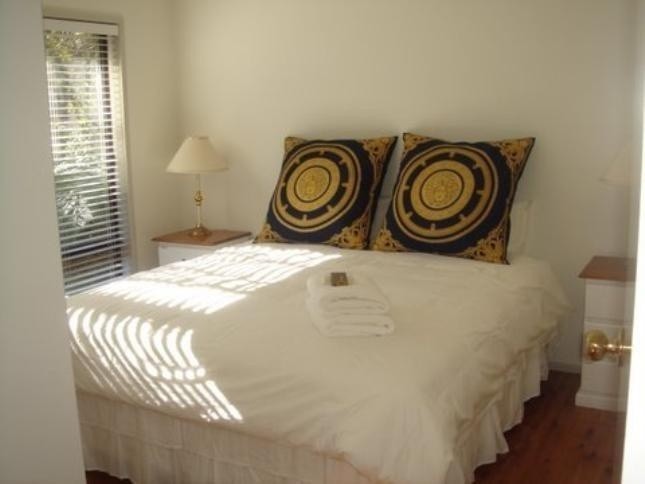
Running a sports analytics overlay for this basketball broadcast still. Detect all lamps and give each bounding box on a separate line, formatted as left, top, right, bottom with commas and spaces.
164, 136, 230, 238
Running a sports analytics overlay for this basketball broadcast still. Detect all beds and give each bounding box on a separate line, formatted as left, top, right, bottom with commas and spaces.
66, 239, 552, 483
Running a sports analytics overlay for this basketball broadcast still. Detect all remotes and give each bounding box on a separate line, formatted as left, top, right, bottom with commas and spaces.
329, 270, 348, 286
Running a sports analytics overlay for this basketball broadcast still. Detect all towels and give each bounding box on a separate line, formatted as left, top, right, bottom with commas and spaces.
302, 269, 394, 340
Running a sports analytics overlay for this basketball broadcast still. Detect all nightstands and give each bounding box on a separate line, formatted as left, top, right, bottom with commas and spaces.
574, 256, 636, 413
150, 227, 252, 266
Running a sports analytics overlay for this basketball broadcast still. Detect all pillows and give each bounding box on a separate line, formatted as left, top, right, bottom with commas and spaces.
252, 132, 536, 266
